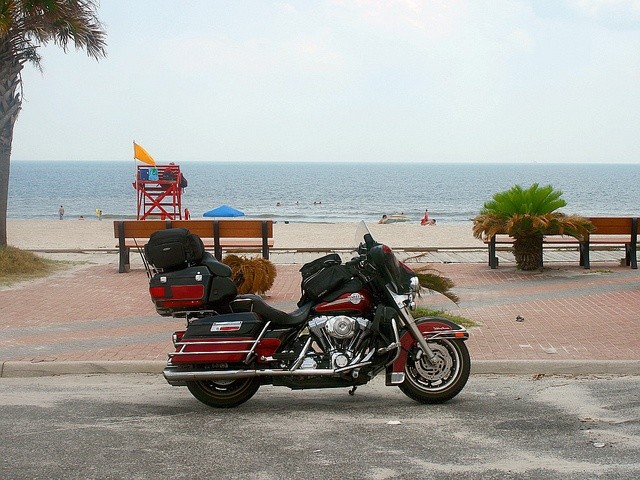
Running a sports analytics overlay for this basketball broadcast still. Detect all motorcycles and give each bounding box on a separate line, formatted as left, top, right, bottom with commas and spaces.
132, 220, 471, 408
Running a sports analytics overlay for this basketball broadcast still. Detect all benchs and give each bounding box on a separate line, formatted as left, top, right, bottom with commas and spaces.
138, 164, 181, 221
488, 215, 640, 271
113, 219, 274, 274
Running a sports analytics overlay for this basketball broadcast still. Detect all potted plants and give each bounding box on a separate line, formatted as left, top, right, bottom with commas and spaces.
471, 181, 598, 270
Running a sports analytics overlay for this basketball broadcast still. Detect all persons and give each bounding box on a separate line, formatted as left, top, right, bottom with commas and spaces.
58, 205, 64, 220
424, 209, 429, 220
422, 219, 436, 226
79, 215, 85, 221
97, 210, 102, 219
378, 215, 388, 225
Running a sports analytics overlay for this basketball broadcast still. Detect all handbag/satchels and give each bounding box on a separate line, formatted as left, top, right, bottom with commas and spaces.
144, 228, 205, 271
297, 254, 353, 308
